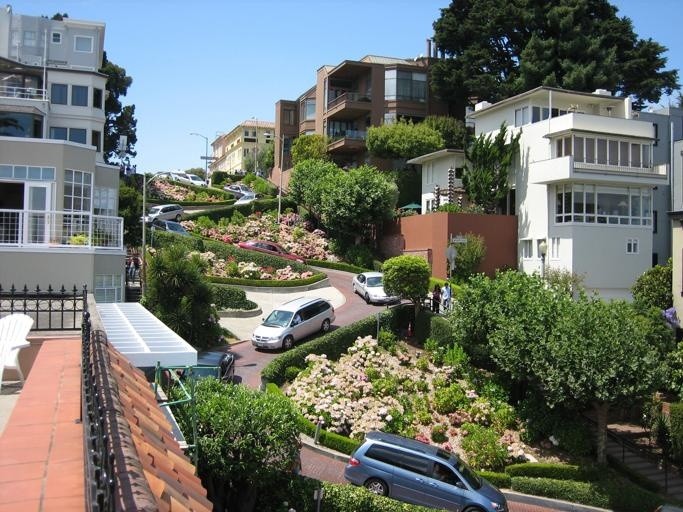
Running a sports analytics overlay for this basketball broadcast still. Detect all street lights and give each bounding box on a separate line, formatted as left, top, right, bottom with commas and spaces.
142, 171, 186, 296
264, 132, 285, 224
251, 115, 259, 174
190, 132, 208, 178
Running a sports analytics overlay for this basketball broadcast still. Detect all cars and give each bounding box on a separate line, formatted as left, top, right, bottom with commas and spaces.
234, 192, 259, 205
152, 219, 190, 236
224, 184, 252, 194
176, 173, 207, 187
353, 271, 401, 304
176, 350, 236, 385
238, 239, 307, 264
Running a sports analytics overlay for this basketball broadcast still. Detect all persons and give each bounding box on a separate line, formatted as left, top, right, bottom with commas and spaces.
432, 283, 441, 313
290, 313, 301, 324
441, 282, 452, 310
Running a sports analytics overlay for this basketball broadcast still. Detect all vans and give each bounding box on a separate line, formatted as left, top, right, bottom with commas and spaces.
139, 203, 183, 224
343, 430, 509, 512
252, 296, 336, 351
